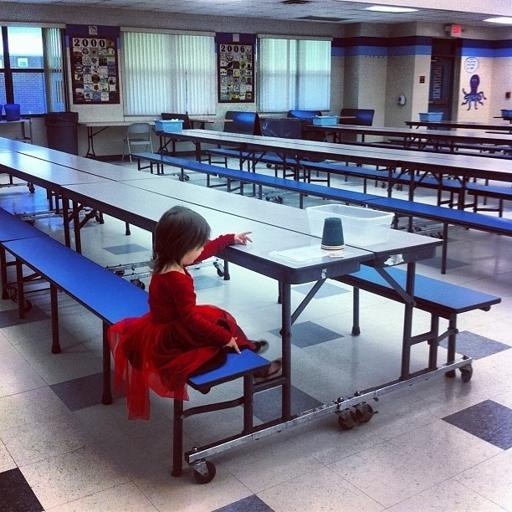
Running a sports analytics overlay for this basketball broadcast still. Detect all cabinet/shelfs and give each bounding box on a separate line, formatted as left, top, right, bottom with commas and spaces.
1, 117, 32, 146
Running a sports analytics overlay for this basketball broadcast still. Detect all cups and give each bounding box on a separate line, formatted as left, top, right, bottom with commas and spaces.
320, 217, 346, 250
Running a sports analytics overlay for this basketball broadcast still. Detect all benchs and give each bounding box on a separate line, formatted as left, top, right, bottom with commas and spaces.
122, 108, 512, 274
1, 207, 271, 479
333, 265, 501, 376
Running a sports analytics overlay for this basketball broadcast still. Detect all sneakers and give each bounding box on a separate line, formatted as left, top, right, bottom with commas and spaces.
250, 339, 268, 354
252, 358, 282, 384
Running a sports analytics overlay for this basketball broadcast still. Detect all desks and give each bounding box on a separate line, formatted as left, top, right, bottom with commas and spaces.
0, 138, 164, 246
61, 176, 473, 484
79, 122, 127, 160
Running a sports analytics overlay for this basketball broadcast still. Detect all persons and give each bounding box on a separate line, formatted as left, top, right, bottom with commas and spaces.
105, 205, 282, 420
